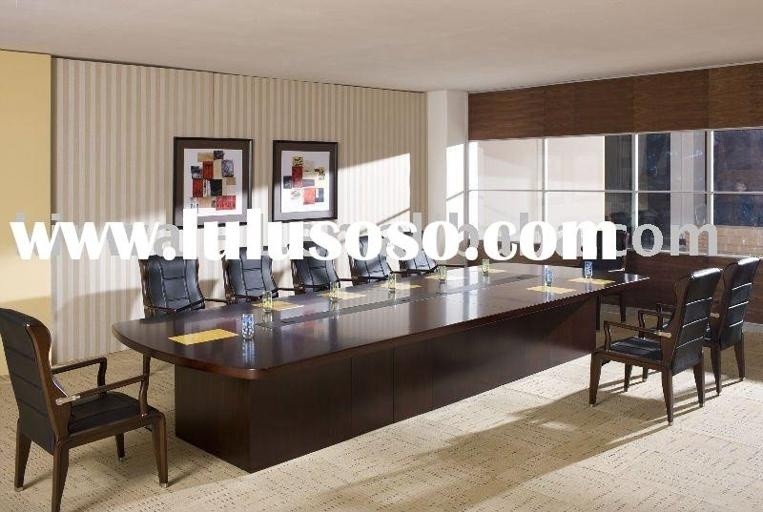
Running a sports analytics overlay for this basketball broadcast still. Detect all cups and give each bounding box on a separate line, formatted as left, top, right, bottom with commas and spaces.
387, 273, 396, 292
545, 271, 552, 285
329, 282, 338, 302
388, 292, 395, 308
328, 303, 339, 320
585, 280, 592, 291
261, 312, 271, 332
436, 266, 446, 281
262, 290, 272, 312
437, 282, 447, 296
239, 336, 256, 363
584, 261, 592, 279
544, 289, 552, 302
481, 259, 489, 273
240, 313, 255, 340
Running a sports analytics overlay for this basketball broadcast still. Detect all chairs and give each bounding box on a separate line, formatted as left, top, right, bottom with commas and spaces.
111, 219, 762, 476
2, 301, 171, 509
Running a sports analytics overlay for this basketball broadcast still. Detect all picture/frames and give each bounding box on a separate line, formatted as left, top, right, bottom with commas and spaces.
172, 135, 252, 228
271, 139, 338, 222
